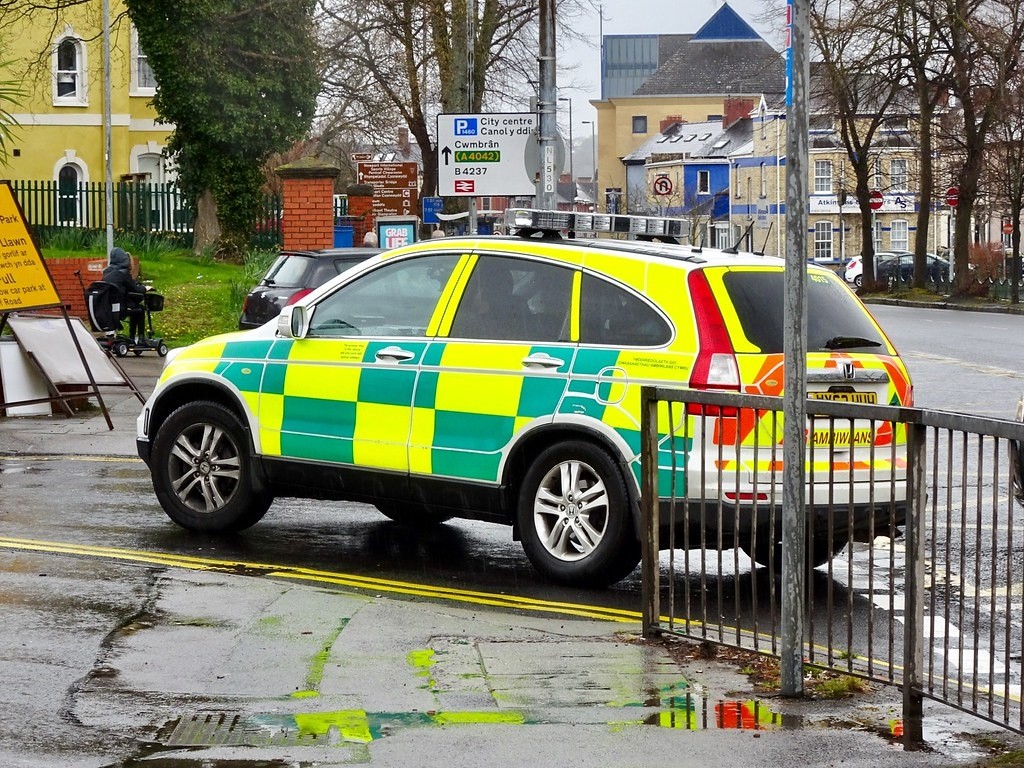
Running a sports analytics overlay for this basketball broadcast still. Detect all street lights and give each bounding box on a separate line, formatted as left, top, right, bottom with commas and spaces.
558, 97, 574, 212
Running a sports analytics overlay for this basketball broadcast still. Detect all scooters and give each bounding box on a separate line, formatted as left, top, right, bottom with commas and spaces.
74, 268, 169, 358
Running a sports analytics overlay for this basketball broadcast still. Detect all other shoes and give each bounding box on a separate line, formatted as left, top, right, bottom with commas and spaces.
138, 338, 146, 346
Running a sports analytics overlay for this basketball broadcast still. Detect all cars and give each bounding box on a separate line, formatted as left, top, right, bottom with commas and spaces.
877, 252, 950, 276
844, 253, 895, 287
883, 249, 912, 258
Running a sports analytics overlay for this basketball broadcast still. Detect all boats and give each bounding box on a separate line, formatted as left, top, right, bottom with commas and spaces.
582, 121, 597, 211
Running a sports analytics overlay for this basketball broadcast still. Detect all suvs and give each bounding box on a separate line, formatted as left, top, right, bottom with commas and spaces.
238, 248, 391, 331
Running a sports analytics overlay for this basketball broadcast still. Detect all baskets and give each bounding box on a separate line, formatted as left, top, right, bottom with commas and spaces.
145, 293, 164, 311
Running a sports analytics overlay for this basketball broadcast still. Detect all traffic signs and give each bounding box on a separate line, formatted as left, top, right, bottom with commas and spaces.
436, 111, 542, 196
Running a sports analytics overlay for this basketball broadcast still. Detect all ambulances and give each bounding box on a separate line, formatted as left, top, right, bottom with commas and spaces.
133, 235, 926, 593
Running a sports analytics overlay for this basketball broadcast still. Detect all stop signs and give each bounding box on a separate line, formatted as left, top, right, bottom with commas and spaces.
946, 188, 959, 207
1002, 224, 1013, 235
868, 190, 884, 210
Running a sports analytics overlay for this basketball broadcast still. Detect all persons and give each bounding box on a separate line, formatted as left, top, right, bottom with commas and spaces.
103, 247, 154, 347
362, 232, 377, 248
